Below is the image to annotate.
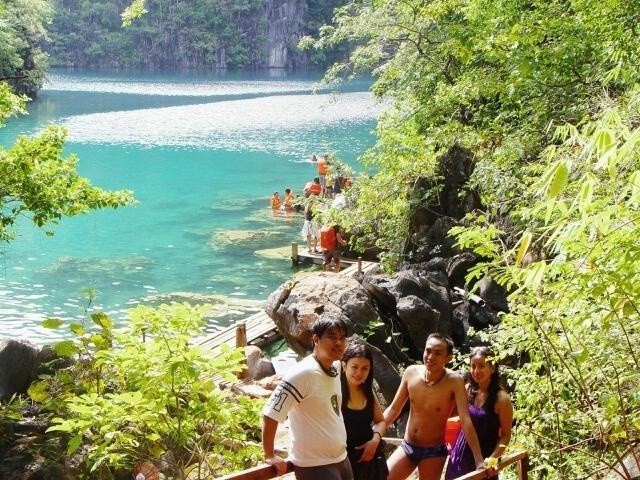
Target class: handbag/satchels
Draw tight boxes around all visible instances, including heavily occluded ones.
[372,440,386,461]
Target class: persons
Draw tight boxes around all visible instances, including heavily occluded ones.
[381,331,499,479]
[261,310,359,480]
[301,204,323,254]
[339,342,391,480]
[269,153,375,213]
[322,223,348,272]
[444,345,515,479]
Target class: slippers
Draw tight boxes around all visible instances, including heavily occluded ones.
[308,249,320,254]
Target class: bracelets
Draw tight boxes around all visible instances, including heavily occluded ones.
[373,432,383,440]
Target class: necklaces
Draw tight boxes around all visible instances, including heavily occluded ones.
[424,368,447,388]
